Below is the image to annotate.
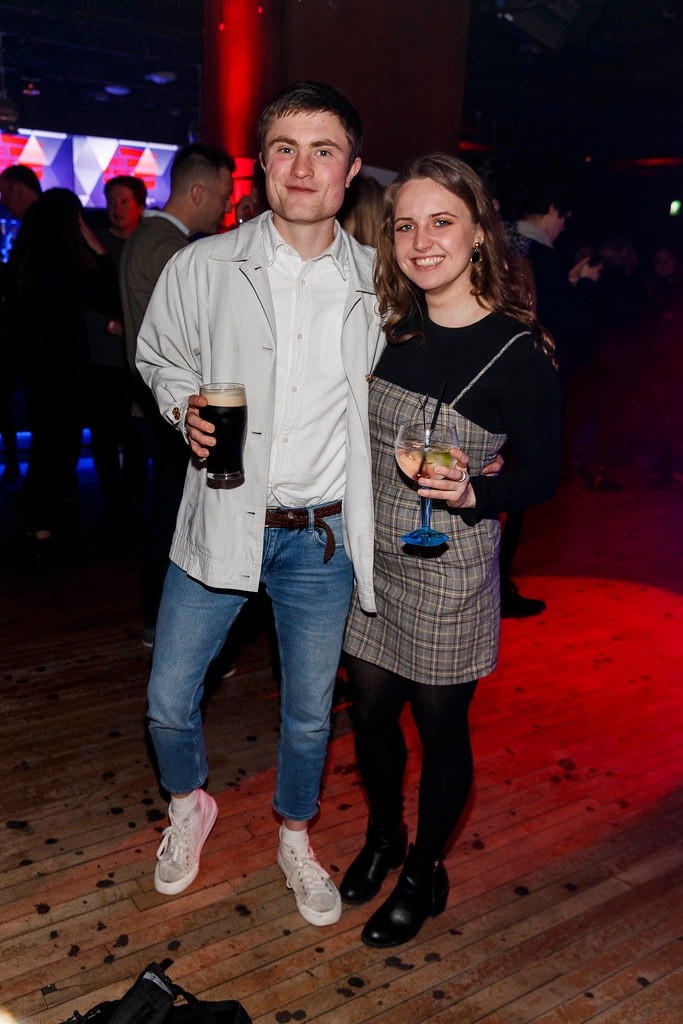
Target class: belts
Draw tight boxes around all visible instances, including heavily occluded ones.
[265,500,342,564]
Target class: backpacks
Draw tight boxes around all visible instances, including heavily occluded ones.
[60,961,253,1024]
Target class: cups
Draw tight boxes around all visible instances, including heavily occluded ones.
[200,382,247,481]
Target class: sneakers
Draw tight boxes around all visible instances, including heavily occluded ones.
[277,826,343,926]
[154,789,218,895]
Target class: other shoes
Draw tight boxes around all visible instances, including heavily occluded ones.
[499,593,545,618]
[143,626,154,647]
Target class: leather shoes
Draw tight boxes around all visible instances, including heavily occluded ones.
[361,841,450,948]
[339,824,408,907]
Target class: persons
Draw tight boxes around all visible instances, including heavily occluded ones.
[337,152,563,950]
[134,81,504,927]
[1,143,682,618]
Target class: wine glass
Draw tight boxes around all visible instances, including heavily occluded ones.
[394,419,459,545]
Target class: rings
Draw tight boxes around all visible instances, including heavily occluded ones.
[458,470,466,484]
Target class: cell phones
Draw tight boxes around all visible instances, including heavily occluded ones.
[587,255,605,266]
